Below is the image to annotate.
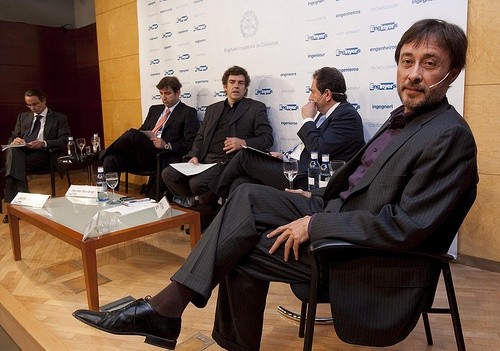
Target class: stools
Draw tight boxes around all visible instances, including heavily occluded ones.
[0,155,71,211]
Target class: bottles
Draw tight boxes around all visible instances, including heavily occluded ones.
[97,166,109,201]
[67,136,77,156]
[307,151,331,190]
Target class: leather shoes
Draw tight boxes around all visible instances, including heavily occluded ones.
[72,295,181,350]
[185,221,211,235]
[172,196,218,214]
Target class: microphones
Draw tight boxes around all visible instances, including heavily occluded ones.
[241,90,246,95]
[40,104,43,110]
[170,97,176,100]
[429,71,451,89]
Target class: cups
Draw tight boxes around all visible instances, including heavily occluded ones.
[330,161,345,176]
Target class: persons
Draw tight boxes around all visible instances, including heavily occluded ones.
[0,87,70,223]
[161,64,274,234]
[72,18,480,350]
[81,76,200,190]
[173,67,366,216]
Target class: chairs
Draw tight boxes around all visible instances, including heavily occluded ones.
[300,186,477,351]
[123,150,173,201]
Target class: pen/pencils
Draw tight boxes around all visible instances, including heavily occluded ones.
[266,149,271,155]
[120,195,151,203]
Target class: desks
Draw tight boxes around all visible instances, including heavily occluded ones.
[54,152,100,190]
[7,190,201,314]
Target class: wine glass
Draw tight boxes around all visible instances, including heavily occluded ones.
[105,172,120,204]
[283,159,298,190]
[76,137,100,157]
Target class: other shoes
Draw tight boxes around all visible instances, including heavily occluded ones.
[4,214,21,223]
[5,178,25,203]
[82,151,101,163]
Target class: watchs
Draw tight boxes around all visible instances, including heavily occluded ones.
[164,142,169,150]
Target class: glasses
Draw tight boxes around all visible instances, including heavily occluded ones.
[25,102,41,107]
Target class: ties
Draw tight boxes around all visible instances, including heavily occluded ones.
[25,115,43,143]
[152,109,170,137]
